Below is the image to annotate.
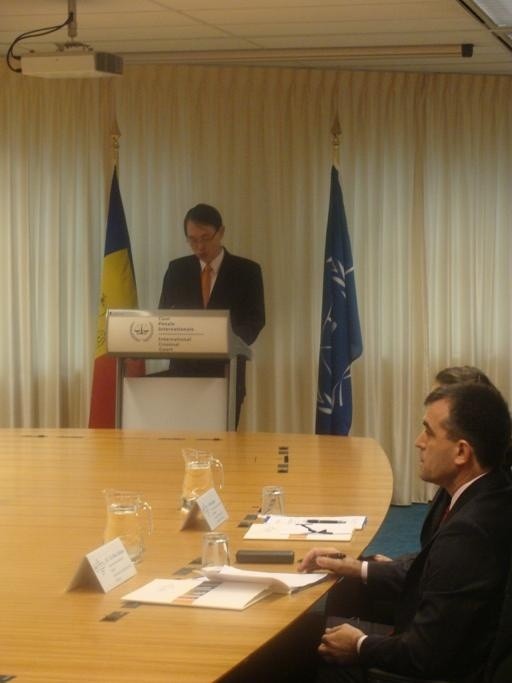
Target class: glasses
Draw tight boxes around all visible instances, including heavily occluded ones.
[183,228,218,249]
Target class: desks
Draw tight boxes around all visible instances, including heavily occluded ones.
[0,425,395,682]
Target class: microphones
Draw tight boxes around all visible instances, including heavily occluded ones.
[169,256,200,309]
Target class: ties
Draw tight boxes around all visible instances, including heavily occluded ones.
[200,264,214,307]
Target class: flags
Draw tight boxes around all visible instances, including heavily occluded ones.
[87,162,146,429]
[315,165,363,436]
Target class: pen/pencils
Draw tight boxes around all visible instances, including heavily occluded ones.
[307,519,346,523]
[297,552,347,563]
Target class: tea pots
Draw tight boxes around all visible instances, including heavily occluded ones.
[181,446,225,516]
[100,486,154,566]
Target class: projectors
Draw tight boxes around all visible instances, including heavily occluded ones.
[21,50,124,79]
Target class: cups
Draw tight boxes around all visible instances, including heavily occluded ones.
[261,484,286,519]
[203,529,230,572]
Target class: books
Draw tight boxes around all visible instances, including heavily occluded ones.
[120,512,367,612]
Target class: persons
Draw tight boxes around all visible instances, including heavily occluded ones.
[156,202,265,433]
[296,384,512,683]
[361,365,497,572]
